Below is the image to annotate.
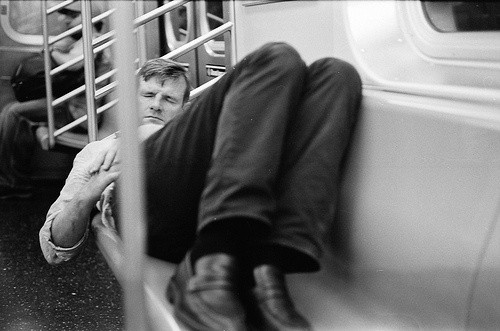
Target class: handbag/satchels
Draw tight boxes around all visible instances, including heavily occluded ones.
[10,52,72,103]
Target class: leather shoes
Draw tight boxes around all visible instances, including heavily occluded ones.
[166,242,250,331]
[252,264,315,331]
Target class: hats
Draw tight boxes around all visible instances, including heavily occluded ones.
[57,1,103,21]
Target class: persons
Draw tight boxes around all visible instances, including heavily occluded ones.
[39,41,363,331]
[0,0,108,198]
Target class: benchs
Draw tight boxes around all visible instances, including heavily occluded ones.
[35,90,117,150]
[92,82,500,330]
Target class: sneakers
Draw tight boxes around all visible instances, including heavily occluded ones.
[0,179,32,200]
